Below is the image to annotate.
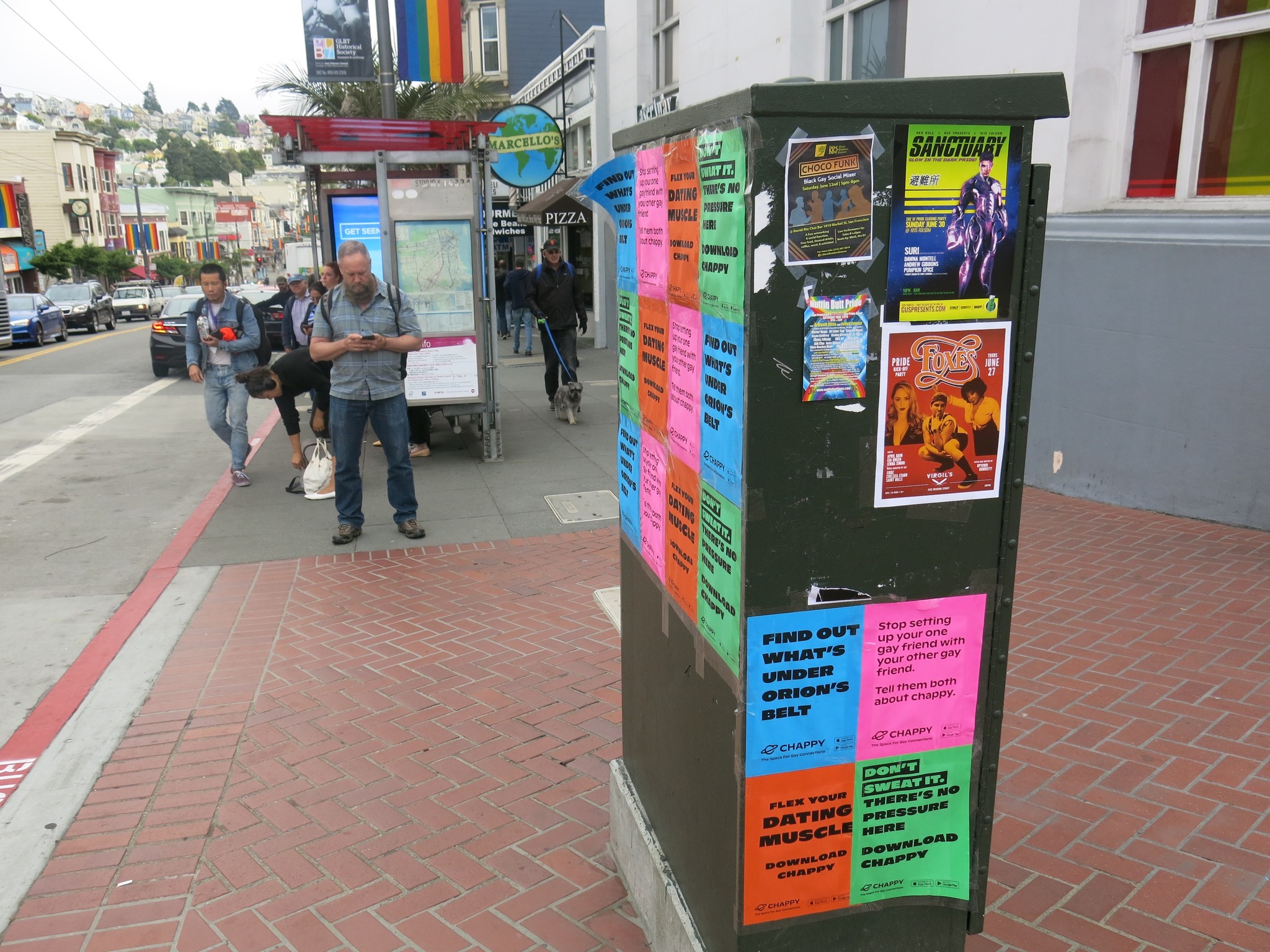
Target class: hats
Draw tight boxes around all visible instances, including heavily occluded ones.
[288,274,304,284]
[543,239,560,251]
[276,276,287,284]
[514,257,524,267]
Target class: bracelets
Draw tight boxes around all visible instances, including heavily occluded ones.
[379,336,387,350]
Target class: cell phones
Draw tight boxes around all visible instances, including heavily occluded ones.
[302,325,309,331]
[362,335,375,340]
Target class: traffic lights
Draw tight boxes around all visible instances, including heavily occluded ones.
[256,256,262,265]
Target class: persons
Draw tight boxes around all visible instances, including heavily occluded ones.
[886,379,1000,489]
[947,153,1006,296]
[309,242,424,543]
[185,239,589,486]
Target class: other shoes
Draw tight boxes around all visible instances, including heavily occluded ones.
[500,334,507,340]
[550,399,556,411]
[497,331,500,336]
[514,350,519,354]
[408,447,430,457]
[506,332,511,337]
[525,351,531,356]
[372,441,383,447]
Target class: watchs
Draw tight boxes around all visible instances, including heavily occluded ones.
[933,429,939,432]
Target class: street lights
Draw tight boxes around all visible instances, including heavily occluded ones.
[203,199,219,260]
[132,160,152,280]
[256,214,267,277]
[235,214,249,285]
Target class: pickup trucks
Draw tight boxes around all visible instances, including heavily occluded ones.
[111,279,165,323]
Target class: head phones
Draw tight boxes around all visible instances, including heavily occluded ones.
[245,379,277,391]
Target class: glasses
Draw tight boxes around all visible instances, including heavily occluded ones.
[549,250,559,254]
[310,297,320,301]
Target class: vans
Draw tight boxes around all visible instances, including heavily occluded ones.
[284,238,322,282]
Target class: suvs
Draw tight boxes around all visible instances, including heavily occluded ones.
[45,279,117,334]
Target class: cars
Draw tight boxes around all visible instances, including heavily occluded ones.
[7,292,68,348]
[149,293,272,378]
[153,284,188,306]
[186,283,287,349]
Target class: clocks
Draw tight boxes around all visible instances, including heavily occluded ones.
[72,200,87,216]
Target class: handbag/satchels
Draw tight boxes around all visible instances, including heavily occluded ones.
[303,437,332,494]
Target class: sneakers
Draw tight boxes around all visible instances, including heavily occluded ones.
[231,470,252,487]
[397,519,425,539]
[331,524,362,545]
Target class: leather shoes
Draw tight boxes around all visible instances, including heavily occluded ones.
[304,491,337,499]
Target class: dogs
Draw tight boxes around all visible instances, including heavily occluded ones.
[554,381,584,425]
